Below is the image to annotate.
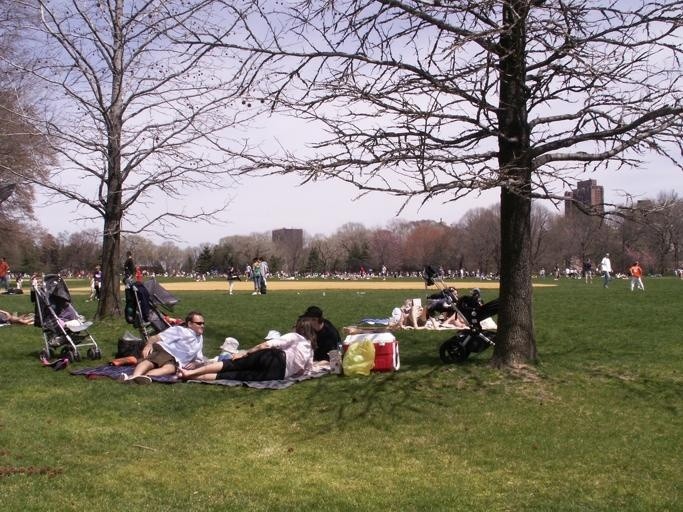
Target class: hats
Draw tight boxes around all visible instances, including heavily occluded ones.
[298,306,322,317]
[265,329,280,341]
[220,338,239,354]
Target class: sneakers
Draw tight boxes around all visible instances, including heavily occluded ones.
[119,372,151,386]
[251,292,261,295]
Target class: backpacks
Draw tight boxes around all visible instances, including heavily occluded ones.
[254,265,260,276]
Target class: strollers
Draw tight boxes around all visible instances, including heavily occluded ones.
[123,277,185,342]
[30,274,100,364]
[420,263,499,364]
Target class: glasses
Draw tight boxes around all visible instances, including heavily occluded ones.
[193,321,206,326]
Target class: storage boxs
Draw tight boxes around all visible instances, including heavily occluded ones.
[343,332,400,372]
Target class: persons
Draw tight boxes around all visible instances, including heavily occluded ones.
[539,266,545,278]
[380,264,387,281]
[176,316,319,383]
[304,305,340,366]
[388,287,484,329]
[244,257,269,295]
[89,263,102,301]
[553,256,593,285]
[628,261,644,291]
[600,252,614,289]
[115,310,206,385]
[124,250,142,289]
[0,257,39,292]
[227,267,237,295]
